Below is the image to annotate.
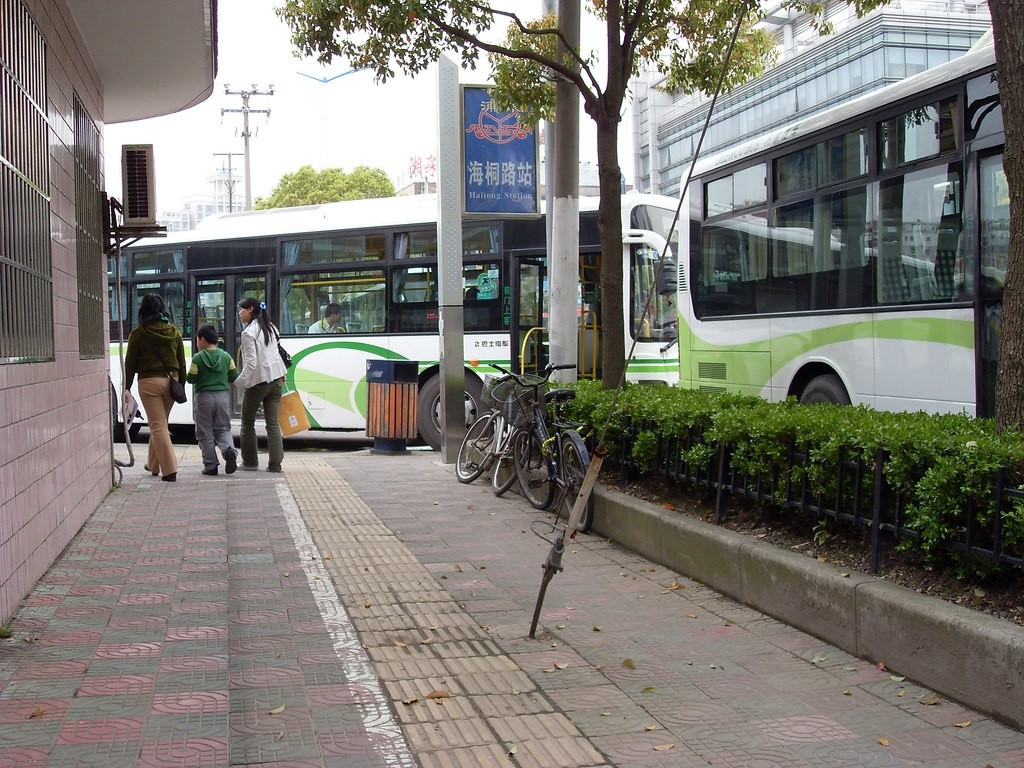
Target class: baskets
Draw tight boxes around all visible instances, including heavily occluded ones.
[480,374,514,408]
[490,377,546,428]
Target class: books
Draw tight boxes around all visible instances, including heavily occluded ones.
[119,390,138,429]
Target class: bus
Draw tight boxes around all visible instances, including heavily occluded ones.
[674,32,1015,421]
[107,188,683,448]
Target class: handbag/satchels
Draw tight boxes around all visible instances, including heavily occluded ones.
[279,383,311,438]
[270,323,292,368]
[170,375,187,404]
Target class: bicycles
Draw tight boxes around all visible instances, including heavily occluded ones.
[487,361,594,536]
[455,374,539,497]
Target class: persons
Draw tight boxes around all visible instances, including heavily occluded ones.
[466,289,477,300]
[307,304,342,333]
[126,292,186,482]
[186,325,238,475]
[231,297,288,472]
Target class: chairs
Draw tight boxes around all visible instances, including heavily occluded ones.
[293,323,310,334]
[169,304,243,334]
[860,232,868,265]
[373,325,385,332]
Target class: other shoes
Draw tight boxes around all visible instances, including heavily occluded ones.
[202,467,218,475]
[225,446,237,474]
[162,472,176,482]
[266,465,281,472]
[236,463,259,471]
[144,463,158,476]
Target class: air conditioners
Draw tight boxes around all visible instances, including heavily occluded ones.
[120,143,158,225]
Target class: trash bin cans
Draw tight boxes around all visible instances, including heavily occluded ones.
[364,358,421,455]
